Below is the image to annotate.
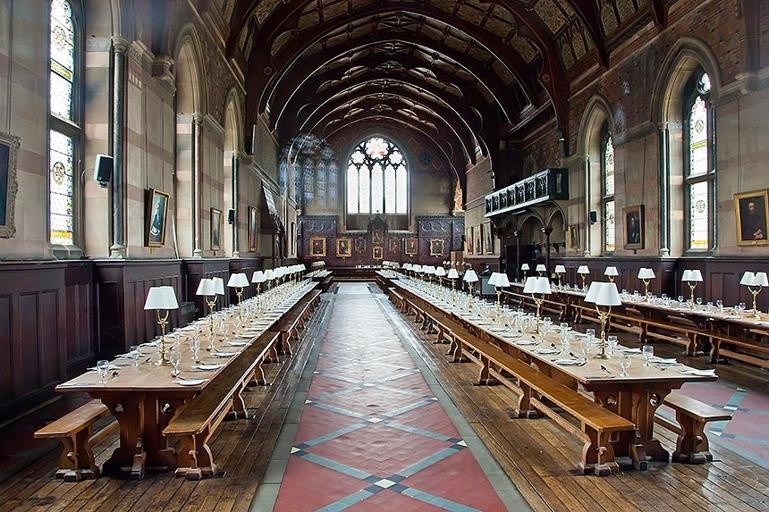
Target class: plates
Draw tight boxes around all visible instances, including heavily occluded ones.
[508,280,769,328]
[394,280,676,367]
[88,281,316,385]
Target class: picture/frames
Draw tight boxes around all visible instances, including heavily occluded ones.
[733,188,769,247]
[404,237,418,255]
[484,220,494,254]
[0,131,23,239]
[465,226,473,255]
[309,237,326,257]
[568,223,580,249]
[145,187,170,247]
[429,239,445,257]
[335,238,352,258]
[248,206,260,252]
[622,204,645,250]
[474,223,482,255]
[211,207,222,251]
[372,246,383,259]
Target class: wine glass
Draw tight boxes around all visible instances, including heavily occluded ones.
[513,278,761,322]
[97,277,312,387]
[398,275,654,376]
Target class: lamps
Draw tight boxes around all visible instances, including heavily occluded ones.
[143,259,327,366]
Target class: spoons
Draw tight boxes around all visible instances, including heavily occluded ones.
[103,281,311,384]
[402,282,669,373]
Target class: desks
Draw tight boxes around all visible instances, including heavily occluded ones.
[325,266,381,278]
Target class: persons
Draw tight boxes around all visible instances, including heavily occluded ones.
[372,229,384,243]
[740,199,768,240]
[406,239,416,254]
[388,238,400,254]
[432,241,443,254]
[626,216,640,244]
[355,239,364,251]
[339,241,348,254]
[151,203,164,235]
[312,241,323,254]
[374,249,381,257]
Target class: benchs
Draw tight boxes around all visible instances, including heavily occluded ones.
[322,276,334,292]
[162,329,281,480]
[34,397,120,482]
[375,275,385,289]
[452,328,636,477]
[653,389,732,464]
